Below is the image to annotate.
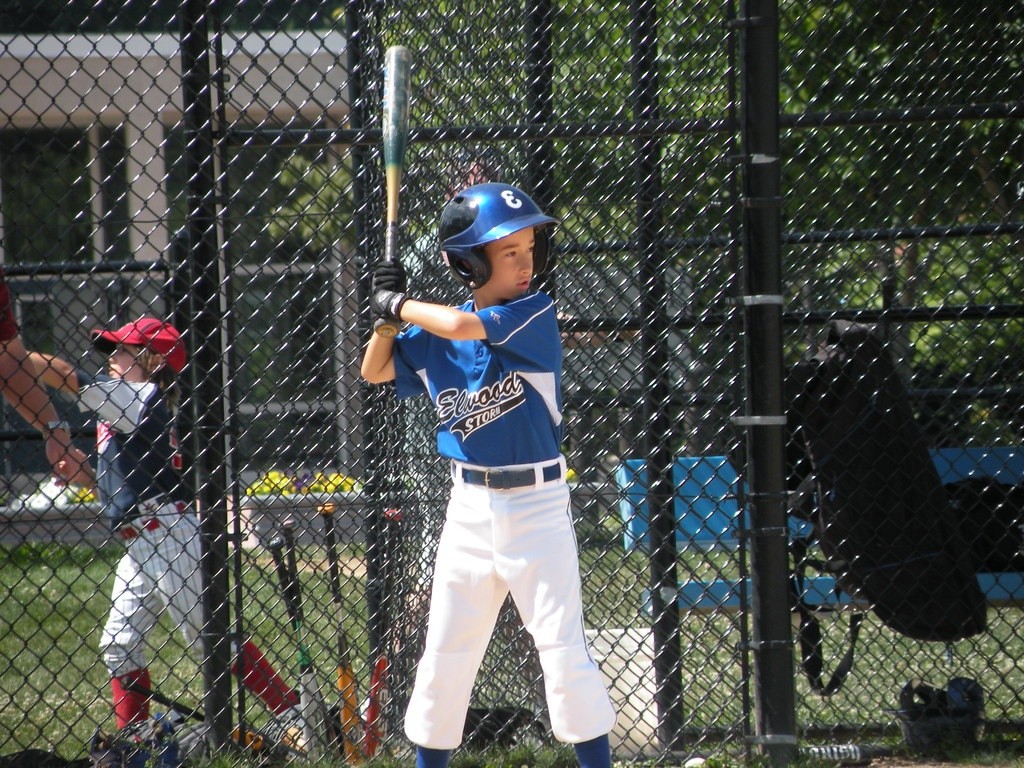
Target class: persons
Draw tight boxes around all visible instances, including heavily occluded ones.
[362,183,618,768]
[27,318,306,750]
[0,278,94,488]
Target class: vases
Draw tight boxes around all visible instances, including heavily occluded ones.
[0,502,113,546]
[236,496,424,546]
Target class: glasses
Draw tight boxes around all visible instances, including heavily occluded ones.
[116,345,136,359]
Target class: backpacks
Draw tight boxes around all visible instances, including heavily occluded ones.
[778,326,989,695]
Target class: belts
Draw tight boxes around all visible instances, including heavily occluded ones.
[120,503,187,540]
[453,463,561,490]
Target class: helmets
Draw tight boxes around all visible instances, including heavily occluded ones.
[891,677,987,760]
[438,182,560,289]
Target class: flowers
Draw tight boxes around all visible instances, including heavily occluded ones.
[12,478,95,510]
[246,470,414,496]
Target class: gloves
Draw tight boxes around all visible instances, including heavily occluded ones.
[371,259,407,322]
[1,265,16,342]
[370,289,413,322]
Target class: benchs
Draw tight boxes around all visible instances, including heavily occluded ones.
[616,446,1024,616]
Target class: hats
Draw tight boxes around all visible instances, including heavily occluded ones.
[91,318,186,375]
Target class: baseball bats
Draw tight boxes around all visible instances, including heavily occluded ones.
[116,675,291,763]
[798,743,876,767]
[266,515,343,767]
[315,501,367,767]
[362,508,407,760]
[372,43,415,340]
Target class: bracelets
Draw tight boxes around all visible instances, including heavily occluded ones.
[44,421,71,437]
